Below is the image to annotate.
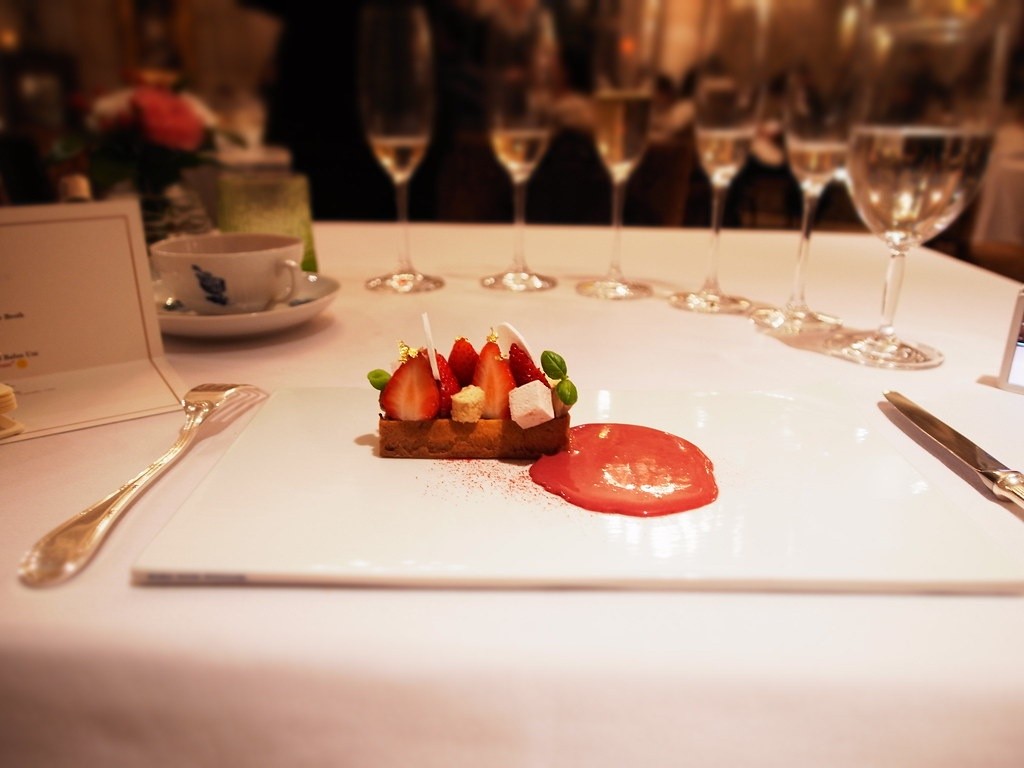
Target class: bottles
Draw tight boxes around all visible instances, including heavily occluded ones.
[214,147,318,273]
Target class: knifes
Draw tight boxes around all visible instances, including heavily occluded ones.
[882,389,1024,510]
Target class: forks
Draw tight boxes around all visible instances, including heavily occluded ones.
[17,382,271,591]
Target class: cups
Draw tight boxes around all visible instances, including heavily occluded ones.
[149,233,304,314]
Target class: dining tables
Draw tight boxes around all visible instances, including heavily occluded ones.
[0,220,1024,768]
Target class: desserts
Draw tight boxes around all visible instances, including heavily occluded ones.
[367,312,578,459]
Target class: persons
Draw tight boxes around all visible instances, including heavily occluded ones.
[0,0,992,262]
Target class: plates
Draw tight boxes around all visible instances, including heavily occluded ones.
[132,365,1022,600]
[151,271,341,337]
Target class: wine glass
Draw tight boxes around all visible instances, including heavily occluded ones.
[575,0,664,302]
[481,2,557,290]
[355,3,444,294]
[664,0,1011,369]
